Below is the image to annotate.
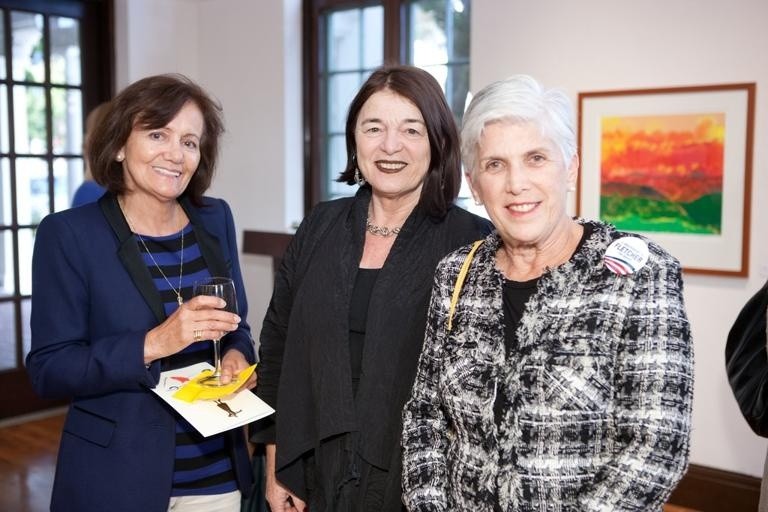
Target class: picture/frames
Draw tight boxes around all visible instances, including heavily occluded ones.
[575,82,754,278]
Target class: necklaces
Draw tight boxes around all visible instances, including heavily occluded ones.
[366,217,402,237]
[118,195,186,308]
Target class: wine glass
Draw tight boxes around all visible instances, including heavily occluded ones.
[192,277,239,388]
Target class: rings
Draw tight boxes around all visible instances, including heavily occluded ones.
[193,329,202,340]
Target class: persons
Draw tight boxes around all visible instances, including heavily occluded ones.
[399,74,697,512]
[250,64,496,511]
[25,73,261,510]
[70,104,117,207]
